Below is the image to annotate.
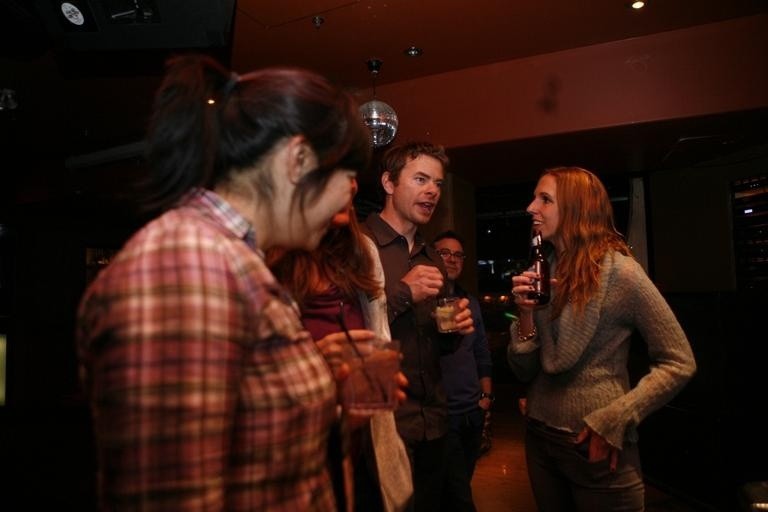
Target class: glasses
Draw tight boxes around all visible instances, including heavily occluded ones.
[437,248,464,260]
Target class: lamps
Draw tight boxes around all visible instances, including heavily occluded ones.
[63,3,149,27]
[352,59,398,147]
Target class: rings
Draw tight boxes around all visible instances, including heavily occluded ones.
[509,289,518,297]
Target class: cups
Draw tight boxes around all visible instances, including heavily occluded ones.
[339,338,402,411]
[433,298,459,335]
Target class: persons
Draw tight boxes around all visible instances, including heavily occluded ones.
[505,166,698,512]
[72,54,410,510]
[353,139,474,511]
[254,138,408,512]
[426,230,495,510]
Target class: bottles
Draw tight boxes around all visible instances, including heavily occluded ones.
[526,230,552,305]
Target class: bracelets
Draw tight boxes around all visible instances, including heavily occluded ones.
[479,392,495,401]
[512,319,537,341]
